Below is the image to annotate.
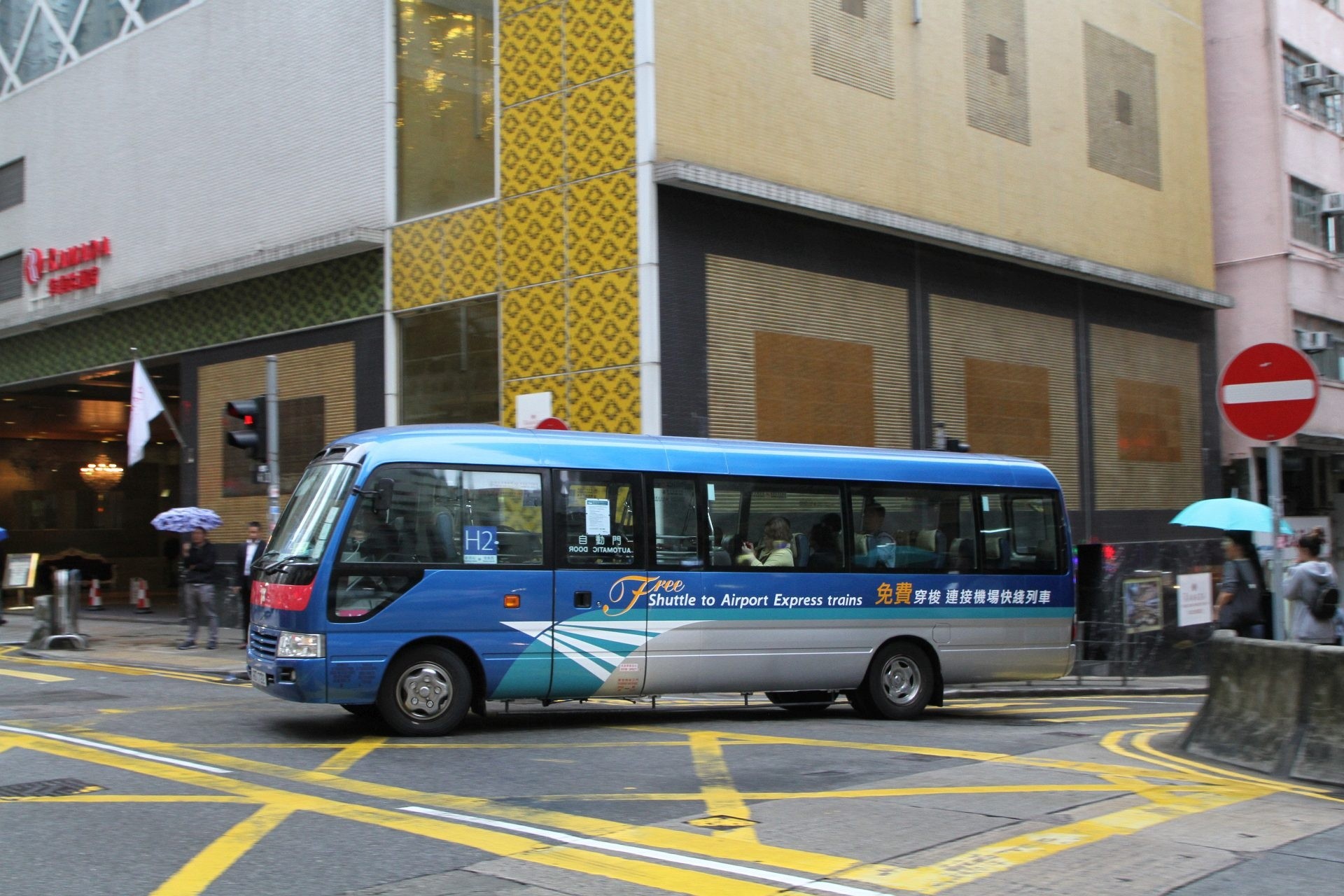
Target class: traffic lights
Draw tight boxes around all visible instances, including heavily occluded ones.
[222,396,264,460]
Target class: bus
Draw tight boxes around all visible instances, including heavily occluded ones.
[239,423,1078,732]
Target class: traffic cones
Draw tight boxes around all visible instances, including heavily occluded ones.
[132,577,153,616]
[87,573,103,610]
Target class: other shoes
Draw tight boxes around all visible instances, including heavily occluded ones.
[239,643,247,650]
[179,639,197,650]
[205,641,218,650]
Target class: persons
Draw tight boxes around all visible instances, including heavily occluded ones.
[345,505,409,592]
[233,521,266,650]
[1212,532,1264,639]
[735,502,896,572]
[177,526,218,650]
[1281,526,1341,644]
[656,527,732,566]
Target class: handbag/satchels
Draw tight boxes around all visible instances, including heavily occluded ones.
[1218,560,1265,630]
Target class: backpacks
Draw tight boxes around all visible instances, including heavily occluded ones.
[1298,583,1340,621]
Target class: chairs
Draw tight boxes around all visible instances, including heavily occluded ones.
[711,519,1051,566]
[427,506,459,561]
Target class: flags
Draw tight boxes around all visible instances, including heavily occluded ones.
[127,359,165,466]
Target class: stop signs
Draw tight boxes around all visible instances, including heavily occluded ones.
[1213,338,1317,447]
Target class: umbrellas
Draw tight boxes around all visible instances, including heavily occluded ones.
[1168,496,1293,535]
[150,506,224,553]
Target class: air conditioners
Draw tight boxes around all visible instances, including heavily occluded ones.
[1318,72,1344,96]
[1299,331,1330,351]
[1323,193,1344,214]
[1299,62,1327,86]
